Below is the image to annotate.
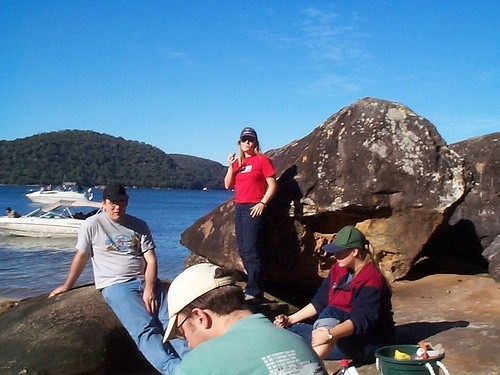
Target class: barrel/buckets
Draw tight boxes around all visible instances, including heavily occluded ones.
[374,345,445,375]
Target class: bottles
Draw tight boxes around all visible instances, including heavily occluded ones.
[336,359,350,375]
[411,348,427,360]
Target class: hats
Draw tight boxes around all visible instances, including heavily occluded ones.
[162,263,235,344]
[322,225,365,252]
[240,127,257,139]
[103,181,129,202]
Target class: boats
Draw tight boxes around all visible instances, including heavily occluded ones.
[0,200,105,237]
[25,182,103,208]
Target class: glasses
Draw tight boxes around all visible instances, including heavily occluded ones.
[105,199,128,207]
[174,310,195,340]
[240,138,255,142]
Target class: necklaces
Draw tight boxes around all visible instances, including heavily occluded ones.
[259,202,266,205]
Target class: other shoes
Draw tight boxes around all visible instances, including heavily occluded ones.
[242,289,258,301]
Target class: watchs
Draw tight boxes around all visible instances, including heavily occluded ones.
[327,330,333,341]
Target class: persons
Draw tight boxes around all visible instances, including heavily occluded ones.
[273,225,395,363]
[162,262,331,375]
[5,207,21,218]
[48,182,192,375]
[224,127,278,299]
